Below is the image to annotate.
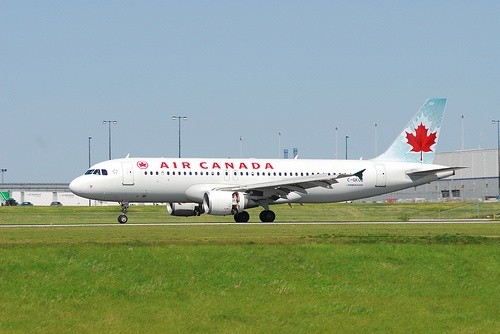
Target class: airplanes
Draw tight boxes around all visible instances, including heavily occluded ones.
[67,98,471,225]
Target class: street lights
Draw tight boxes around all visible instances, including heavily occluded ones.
[1,168,7,184]
[239,137,243,159]
[374,122,378,156]
[335,127,338,158]
[172,116,188,158]
[492,119,500,199]
[344,135,349,160]
[102,121,117,160]
[335,127,339,158]
[88,136,92,168]
[279,132,281,158]
[460,113,464,151]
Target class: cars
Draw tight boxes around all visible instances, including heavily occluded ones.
[50,201,62,206]
[21,201,33,206]
